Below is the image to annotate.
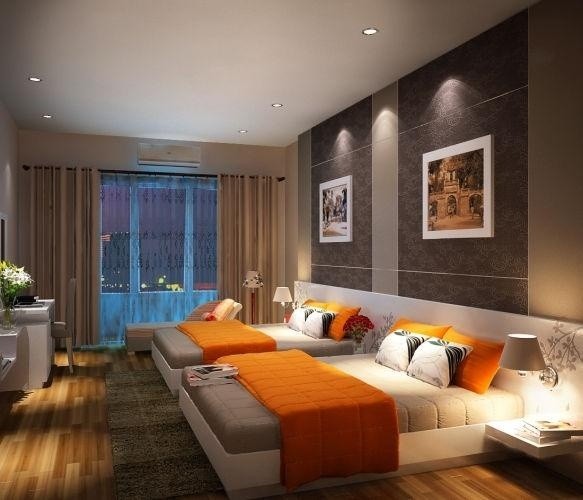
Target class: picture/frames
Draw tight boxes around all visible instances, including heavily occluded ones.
[317,175,354,245]
[420,133,493,241]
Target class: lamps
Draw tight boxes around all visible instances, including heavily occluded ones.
[272,286,299,310]
[240,270,263,326]
[497,332,556,391]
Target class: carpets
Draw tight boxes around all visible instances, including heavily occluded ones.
[101,367,228,500]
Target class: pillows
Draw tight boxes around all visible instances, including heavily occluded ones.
[286,305,315,332]
[373,327,429,372]
[186,298,235,323]
[389,317,451,339]
[302,298,328,309]
[325,301,360,342]
[442,325,505,395]
[302,310,337,338]
[408,334,474,389]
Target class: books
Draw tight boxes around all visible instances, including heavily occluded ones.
[516,415,582,445]
[188,381,233,386]
[187,362,238,379]
[186,371,236,383]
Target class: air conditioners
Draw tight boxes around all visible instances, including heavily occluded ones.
[136,142,202,169]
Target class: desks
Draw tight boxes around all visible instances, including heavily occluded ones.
[0,297,55,390]
[0,327,23,383]
[486,418,582,460]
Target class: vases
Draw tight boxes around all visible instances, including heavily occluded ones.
[352,335,365,354]
[1,305,18,330]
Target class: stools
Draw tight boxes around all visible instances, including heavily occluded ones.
[50,322,76,375]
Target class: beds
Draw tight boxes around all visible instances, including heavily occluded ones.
[175,296,582,500]
[124,294,241,354]
[150,281,378,397]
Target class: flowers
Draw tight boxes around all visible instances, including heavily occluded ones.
[340,312,373,342]
[0,262,34,329]
